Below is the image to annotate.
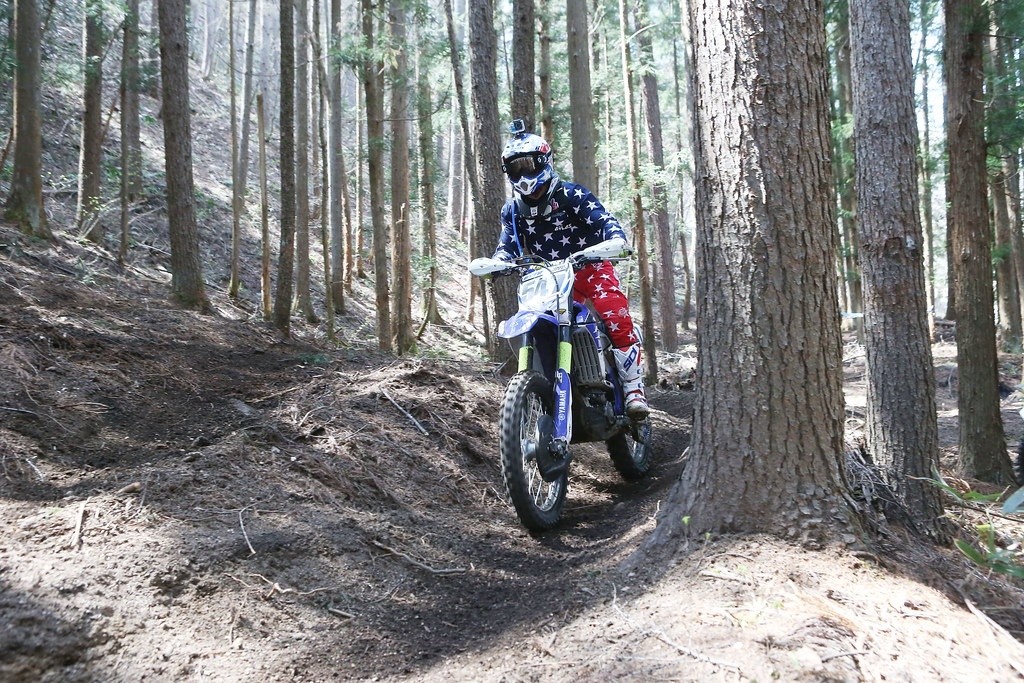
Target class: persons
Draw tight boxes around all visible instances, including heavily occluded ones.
[478,133,651,421]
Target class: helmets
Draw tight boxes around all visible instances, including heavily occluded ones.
[501,132,555,196]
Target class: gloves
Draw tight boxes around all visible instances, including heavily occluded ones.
[609,249,628,267]
[480,273,495,279]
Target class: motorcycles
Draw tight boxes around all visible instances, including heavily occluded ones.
[467,239,654,533]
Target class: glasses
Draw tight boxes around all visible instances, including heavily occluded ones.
[500,147,553,182]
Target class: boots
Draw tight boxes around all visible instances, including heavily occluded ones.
[612,342,650,421]
